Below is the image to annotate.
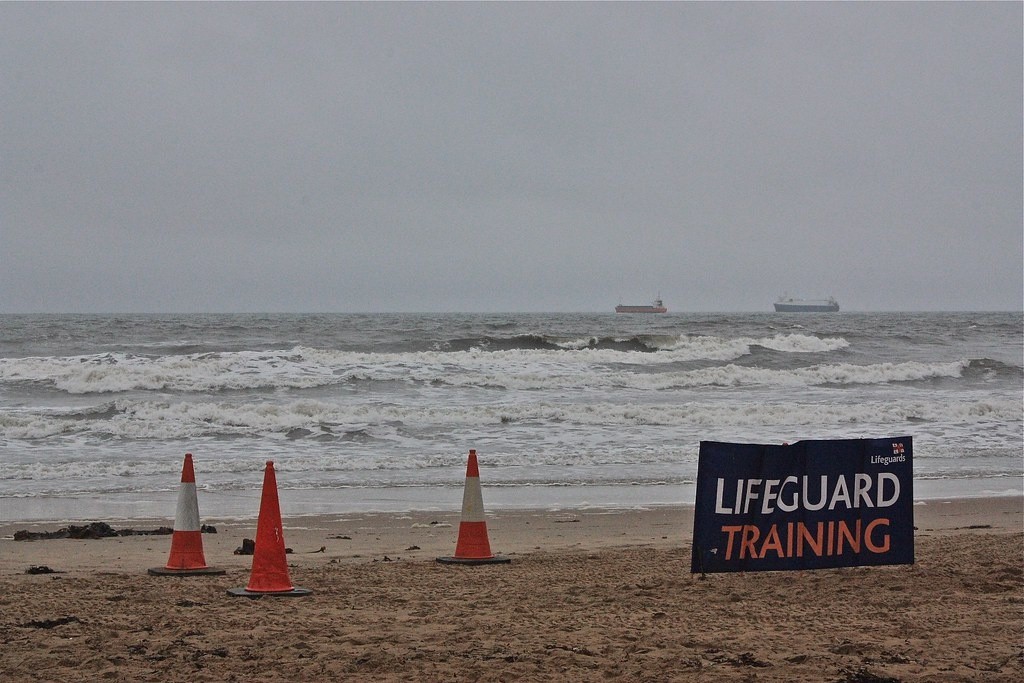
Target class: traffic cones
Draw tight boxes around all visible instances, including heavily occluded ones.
[434,449,512,566]
[227,461,306,598]
[146,453,226,576]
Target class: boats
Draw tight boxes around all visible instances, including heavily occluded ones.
[615,300,669,317]
[773,292,839,313]
